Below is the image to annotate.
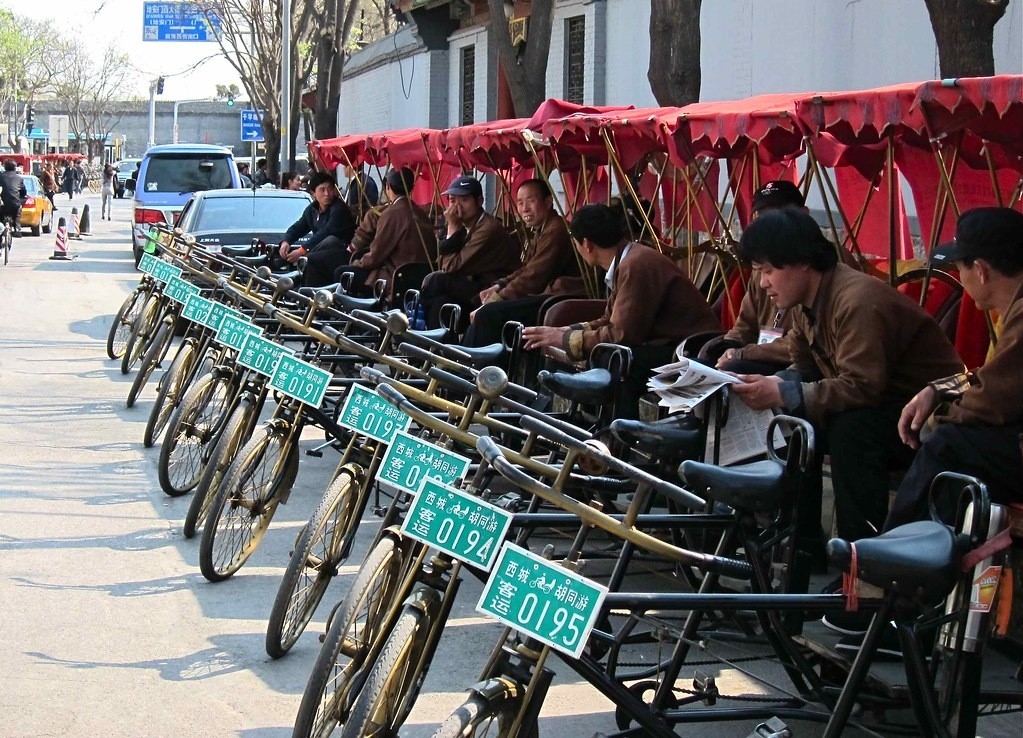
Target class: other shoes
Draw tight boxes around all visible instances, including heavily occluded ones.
[52,207,58,211]
[800,534,827,563]
[13,232,22,237]
[822,611,875,633]
[836,633,904,659]
[108,217,111,221]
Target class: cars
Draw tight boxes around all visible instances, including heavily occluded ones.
[0,173,54,237]
[165,188,320,334]
[113,158,145,198]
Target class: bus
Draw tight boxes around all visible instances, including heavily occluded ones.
[0,127,122,186]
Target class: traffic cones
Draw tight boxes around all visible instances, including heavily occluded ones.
[50,217,78,261]
[66,207,83,240]
[79,204,94,236]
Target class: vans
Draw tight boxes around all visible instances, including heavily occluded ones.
[131,140,242,260]
[234,155,316,194]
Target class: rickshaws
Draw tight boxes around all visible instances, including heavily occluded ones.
[103,100,1019,738]
[0,154,39,177]
[41,153,88,192]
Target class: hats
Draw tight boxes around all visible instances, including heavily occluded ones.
[929,207,1023,266]
[751,179,804,215]
[441,176,481,197]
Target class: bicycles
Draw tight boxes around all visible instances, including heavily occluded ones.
[2,214,11,265]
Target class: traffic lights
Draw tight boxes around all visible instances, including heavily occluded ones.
[226,99,234,106]
[156,77,164,95]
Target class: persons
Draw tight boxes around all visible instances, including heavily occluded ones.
[278,172,356,288]
[822,206,1023,655]
[761,339,768,345]
[101,158,124,221]
[344,160,378,219]
[0,159,27,256]
[237,158,316,192]
[132,161,142,180]
[15,160,86,211]
[338,167,971,598]
